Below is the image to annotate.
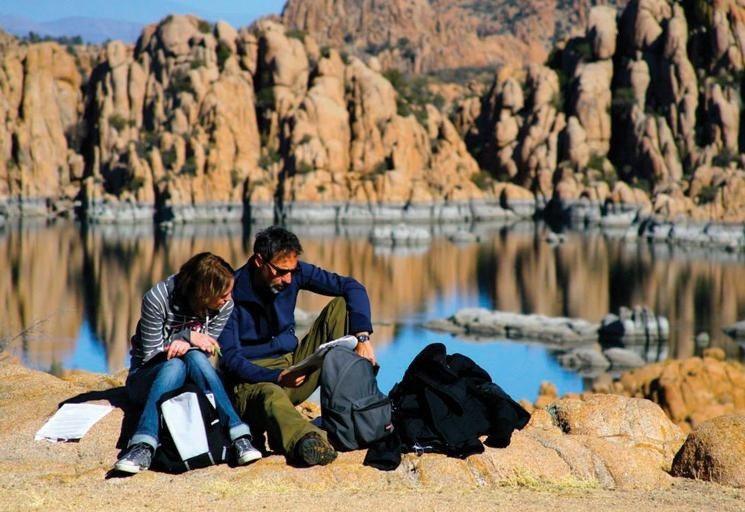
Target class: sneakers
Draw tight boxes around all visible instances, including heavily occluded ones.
[231,438,262,465]
[115,443,152,473]
[298,432,337,465]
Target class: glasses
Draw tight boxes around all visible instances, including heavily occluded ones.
[268,261,296,275]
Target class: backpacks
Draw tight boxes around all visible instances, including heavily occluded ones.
[319,345,395,451]
[156,383,230,474]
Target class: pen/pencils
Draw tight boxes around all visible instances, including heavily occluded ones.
[214,345,223,357]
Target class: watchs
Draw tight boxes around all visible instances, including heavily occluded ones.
[355,334,370,342]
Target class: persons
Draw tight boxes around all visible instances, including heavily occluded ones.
[214,225,374,466]
[113,253,263,473]
